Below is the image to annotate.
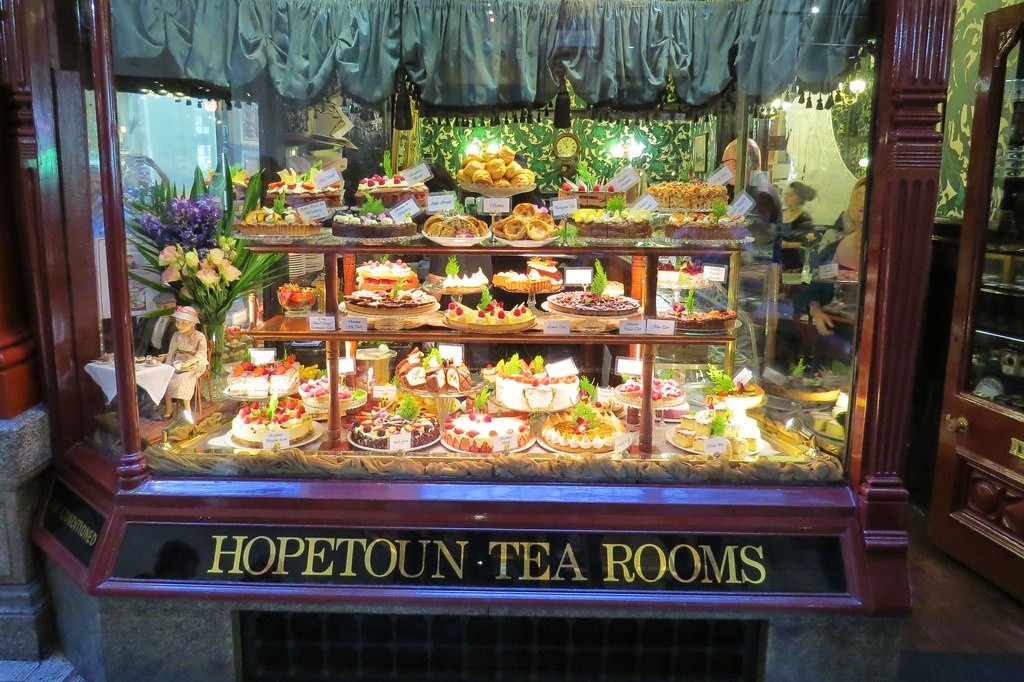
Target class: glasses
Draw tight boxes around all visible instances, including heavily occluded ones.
[720,158,736,165]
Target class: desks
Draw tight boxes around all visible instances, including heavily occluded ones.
[84,359,175,421]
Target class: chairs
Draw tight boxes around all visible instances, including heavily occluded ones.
[195,339,213,412]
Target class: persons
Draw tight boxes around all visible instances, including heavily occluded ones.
[424,164,494,310]
[782,181,816,273]
[720,138,782,358]
[163,306,207,418]
[800,172,867,380]
[462,155,558,358]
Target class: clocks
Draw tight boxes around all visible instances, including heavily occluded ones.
[553,132,580,160]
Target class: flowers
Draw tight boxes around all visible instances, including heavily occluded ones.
[120,165,295,362]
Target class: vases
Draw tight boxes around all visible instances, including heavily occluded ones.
[201,323,230,403]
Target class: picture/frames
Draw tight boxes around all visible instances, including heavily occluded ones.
[693,132,709,173]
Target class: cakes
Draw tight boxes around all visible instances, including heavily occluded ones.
[226,151,839,455]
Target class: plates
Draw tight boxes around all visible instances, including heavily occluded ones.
[492,234,560,247]
[421,229,492,246]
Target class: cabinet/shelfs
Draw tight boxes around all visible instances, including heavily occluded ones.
[242,240,742,453]
[927,3,1024,606]
